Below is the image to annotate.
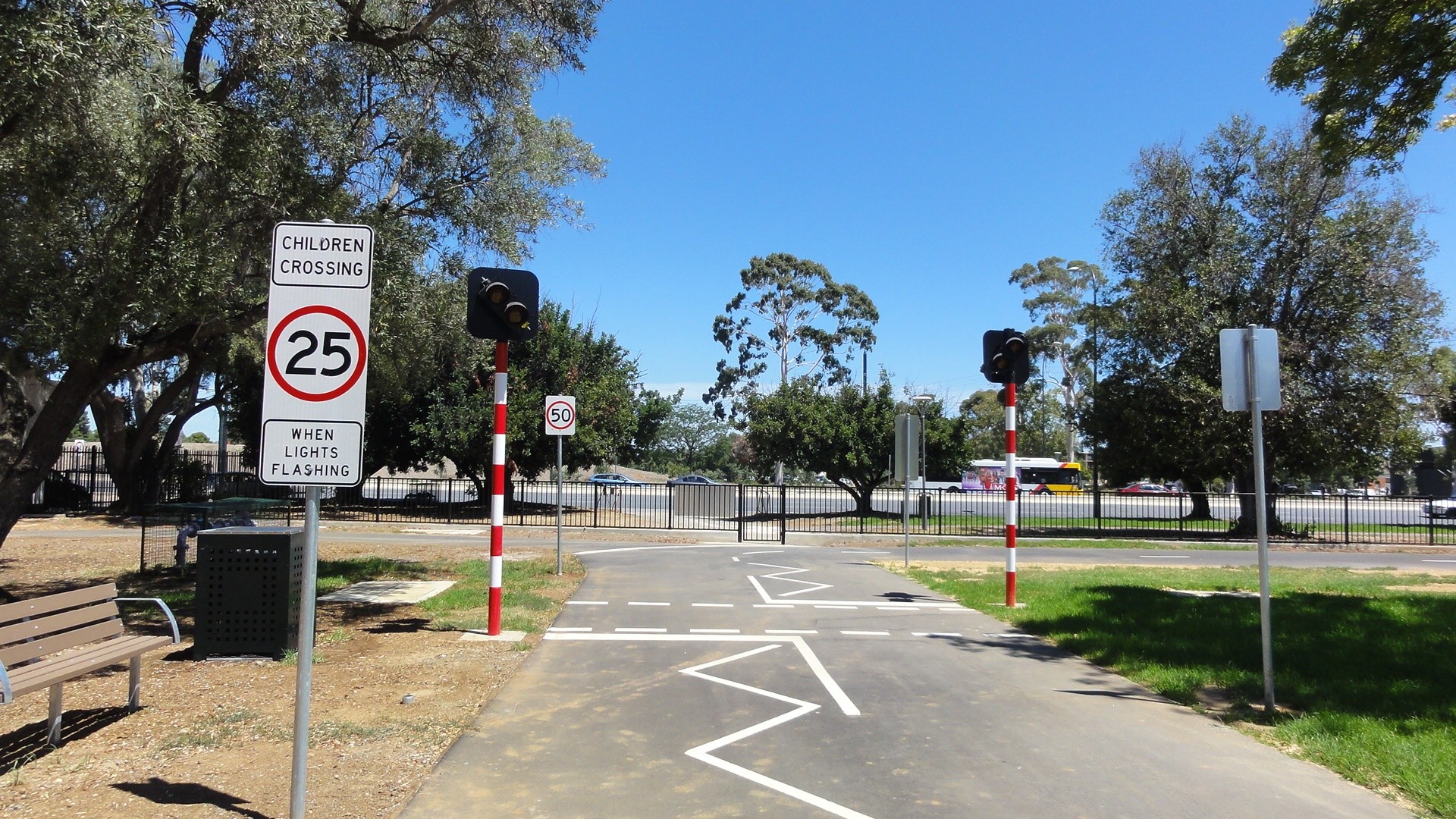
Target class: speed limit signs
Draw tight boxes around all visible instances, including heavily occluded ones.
[267,305,366,402]
[546,395,576,436]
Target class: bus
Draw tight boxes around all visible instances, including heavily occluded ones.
[961,458,1084,496]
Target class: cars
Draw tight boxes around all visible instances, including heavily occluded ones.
[1421,495,1456,519]
[667,475,720,485]
[1117,482,1187,498]
[189,472,293,500]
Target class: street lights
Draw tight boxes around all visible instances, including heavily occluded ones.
[912,397,932,530]
[1067,265,1100,518]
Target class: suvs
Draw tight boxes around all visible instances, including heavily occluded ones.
[586,473,651,487]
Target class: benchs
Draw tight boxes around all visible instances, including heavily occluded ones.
[0,582,180,746]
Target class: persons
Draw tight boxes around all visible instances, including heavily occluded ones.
[1320,483,1325,500]
[985,470,991,489]
[991,470,997,489]
[997,470,1005,484]
[1386,487,1388,496]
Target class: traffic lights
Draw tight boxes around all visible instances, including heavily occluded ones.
[466,267,540,341]
[982,329,1030,385]
